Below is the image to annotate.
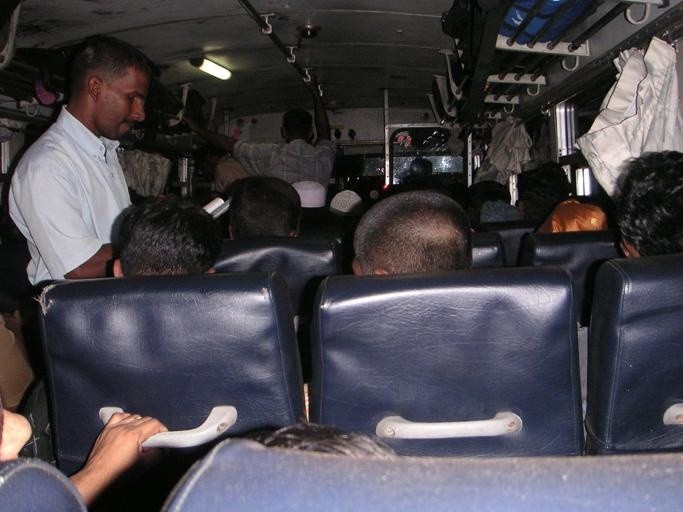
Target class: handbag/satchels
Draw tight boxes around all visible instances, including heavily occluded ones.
[0,45,183,141]
[440,0,580,77]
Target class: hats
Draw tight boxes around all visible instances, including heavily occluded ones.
[331,190,362,216]
[292,181,325,208]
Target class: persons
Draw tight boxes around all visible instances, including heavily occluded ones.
[115,149,683,438]
[181,74,336,193]
[1,304,168,512]
[263,419,399,456]
[9,35,151,286]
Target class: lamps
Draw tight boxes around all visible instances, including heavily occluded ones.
[190,56,232,85]
